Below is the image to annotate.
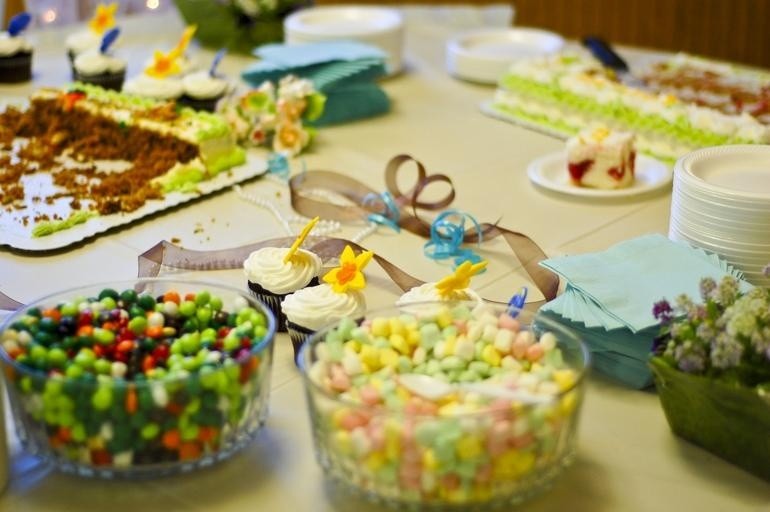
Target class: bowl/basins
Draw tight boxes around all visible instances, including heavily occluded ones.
[1,278,277,481]
[296,301,592,510]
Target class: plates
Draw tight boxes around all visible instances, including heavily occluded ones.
[284,1,407,79]
[670,147,770,289]
[446,28,566,88]
[526,149,672,200]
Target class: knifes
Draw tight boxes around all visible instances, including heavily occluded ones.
[583,34,648,91]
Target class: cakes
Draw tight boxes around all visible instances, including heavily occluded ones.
[280,244,373,369]
[0,82,246,235]
[243,217,321,332]
[183,70,231,109]
[67,34,125,93]
[0,37,36,82]
[496,34,769,161]
[560,124,642,187]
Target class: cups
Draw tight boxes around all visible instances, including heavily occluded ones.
[1,48,32,84]
[72,67,126,94]
[283,316,370,366]
[181,91,227,116]
[248,278,321,334]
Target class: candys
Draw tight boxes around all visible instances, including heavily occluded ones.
[311,302,575,506]
[0,288,267,467]
